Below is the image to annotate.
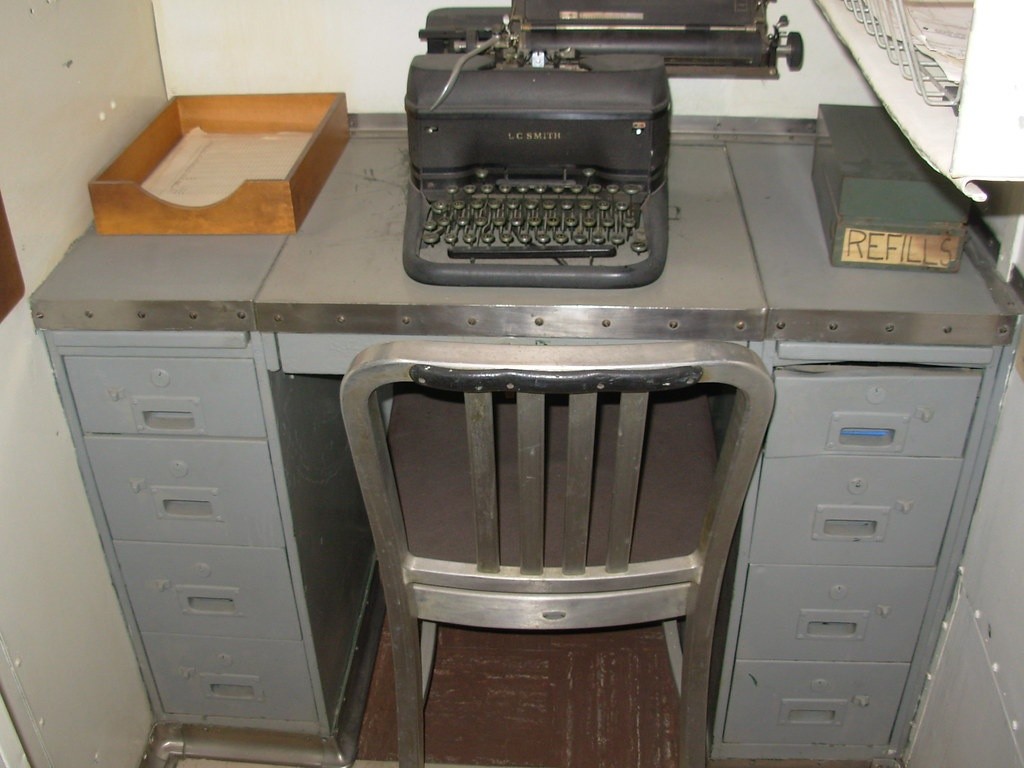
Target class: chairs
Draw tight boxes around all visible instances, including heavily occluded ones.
[338,341,775,768]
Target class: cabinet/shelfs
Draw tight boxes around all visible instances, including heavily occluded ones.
[29,127,1024,768]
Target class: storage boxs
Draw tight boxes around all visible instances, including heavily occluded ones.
[89,94,347,237]
[815,104,969,274]
